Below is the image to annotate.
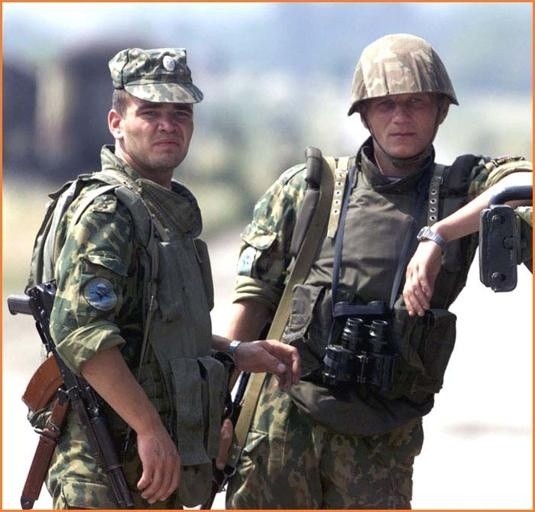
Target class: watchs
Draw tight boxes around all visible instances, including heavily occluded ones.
[414,225,447,254]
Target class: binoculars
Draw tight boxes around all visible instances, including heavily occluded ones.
[323,318,392,401]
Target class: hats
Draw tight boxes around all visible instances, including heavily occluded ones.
[109,48,203,104]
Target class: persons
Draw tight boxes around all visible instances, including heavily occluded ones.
[223,31,532,510]
[21,44,306,509]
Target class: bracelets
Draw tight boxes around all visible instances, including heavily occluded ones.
[226,339,242,356]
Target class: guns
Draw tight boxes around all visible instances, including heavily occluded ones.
[6,281,133,506]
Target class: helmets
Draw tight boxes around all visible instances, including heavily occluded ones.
[348,34,459,116]
